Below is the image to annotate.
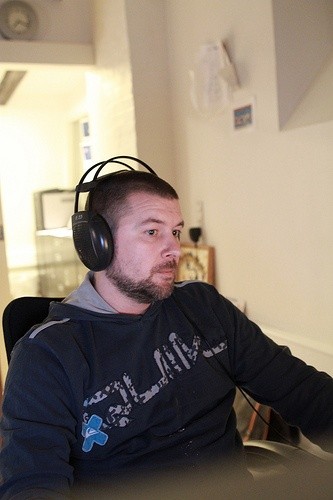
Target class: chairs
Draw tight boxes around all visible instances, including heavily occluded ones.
[3,298,331,500]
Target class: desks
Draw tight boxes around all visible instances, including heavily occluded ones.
[173,240,216,288]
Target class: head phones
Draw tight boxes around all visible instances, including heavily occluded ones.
[71,156,157,271]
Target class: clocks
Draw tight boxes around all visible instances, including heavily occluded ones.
[0,0,40,41]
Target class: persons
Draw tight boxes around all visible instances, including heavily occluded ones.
[1,171,333,500]
